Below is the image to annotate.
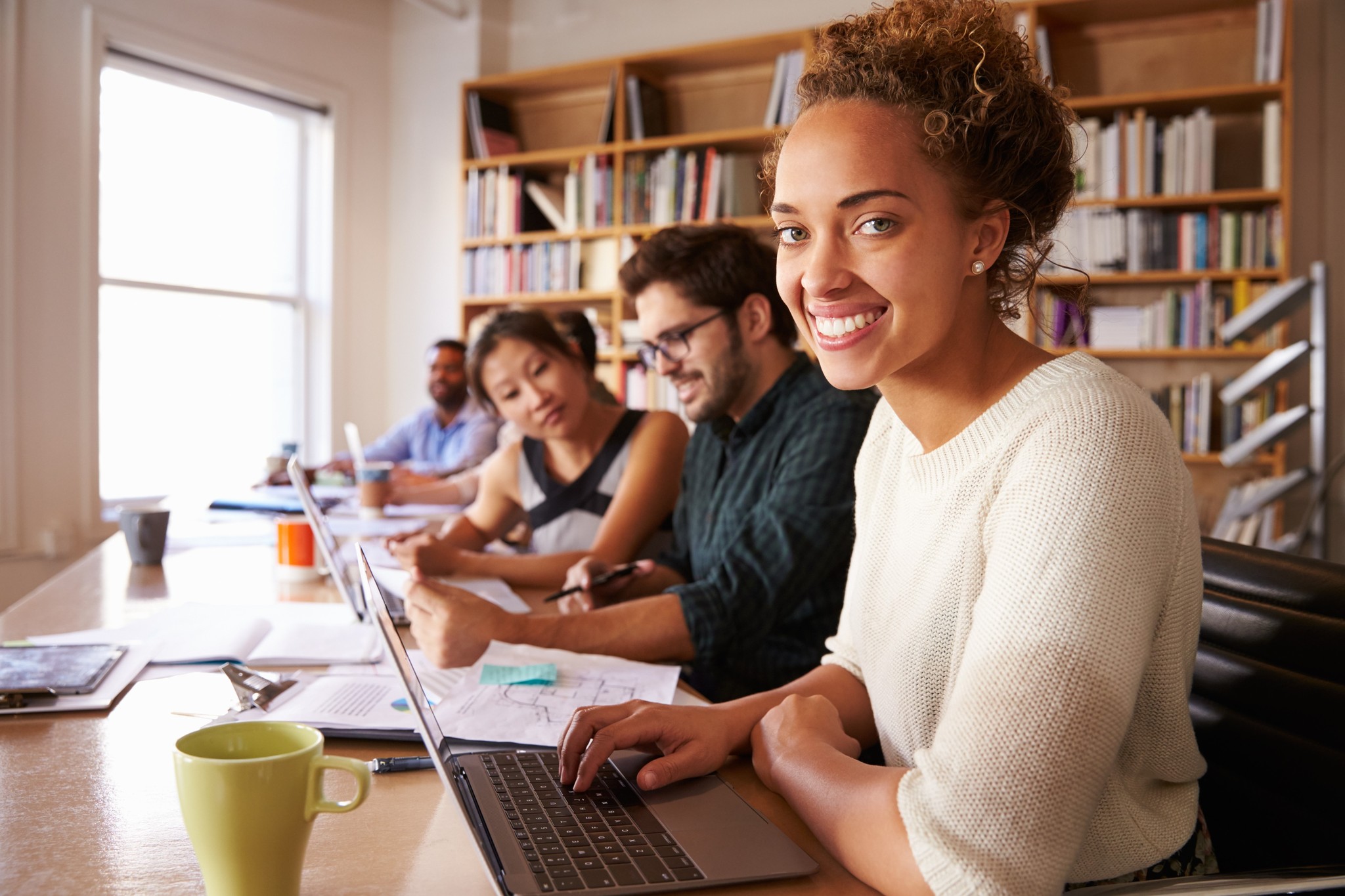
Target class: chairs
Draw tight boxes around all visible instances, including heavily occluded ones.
[1074,531,1344,896]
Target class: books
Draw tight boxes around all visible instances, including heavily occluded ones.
[465,50,815,411]
[1004,0,1286,551]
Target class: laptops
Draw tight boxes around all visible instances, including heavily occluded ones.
[286,455,816,896]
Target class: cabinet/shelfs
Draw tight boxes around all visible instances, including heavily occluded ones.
[458,2,1335,555]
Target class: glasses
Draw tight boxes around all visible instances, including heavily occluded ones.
[637,310,736,369]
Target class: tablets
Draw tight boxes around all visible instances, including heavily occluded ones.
[0,644,129,693]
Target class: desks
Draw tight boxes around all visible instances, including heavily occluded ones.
[0,487,870,896]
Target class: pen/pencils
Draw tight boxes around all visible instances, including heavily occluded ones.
[363,757,435,772]
[543,564,638,601]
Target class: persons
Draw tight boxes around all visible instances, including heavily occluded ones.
[322,309,694,584]
[404,221,879,702]
[556,0,1220,896]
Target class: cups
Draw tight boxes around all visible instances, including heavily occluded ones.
[120,510,170,564]
[274,518,333,584]
[354,461,393,518]
[174,721,370,896]
